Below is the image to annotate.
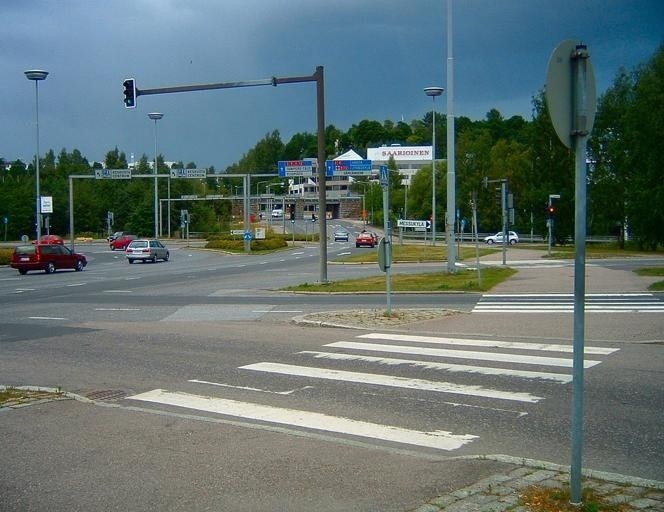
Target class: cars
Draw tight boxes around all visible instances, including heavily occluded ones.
[356,230,379,248]
[32,234,64,245]
[125,239,169,263]
[484,230,519,245]
[334,229,348,241]
[109,236,134,251]
[9,244,87,274]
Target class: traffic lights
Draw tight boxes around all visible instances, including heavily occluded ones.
[122,78,137,110]
[545,206,555,220]
[184,213,190,223]
[290,203,296,223]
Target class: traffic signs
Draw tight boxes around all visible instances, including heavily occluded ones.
[277,159,312,179]
[170,168,207,179]
[95,168,131,179]
[326,159,371,177]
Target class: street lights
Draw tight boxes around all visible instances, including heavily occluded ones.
[23,70,49,241]
[148,111,165,237]
[424,87,444,247]
